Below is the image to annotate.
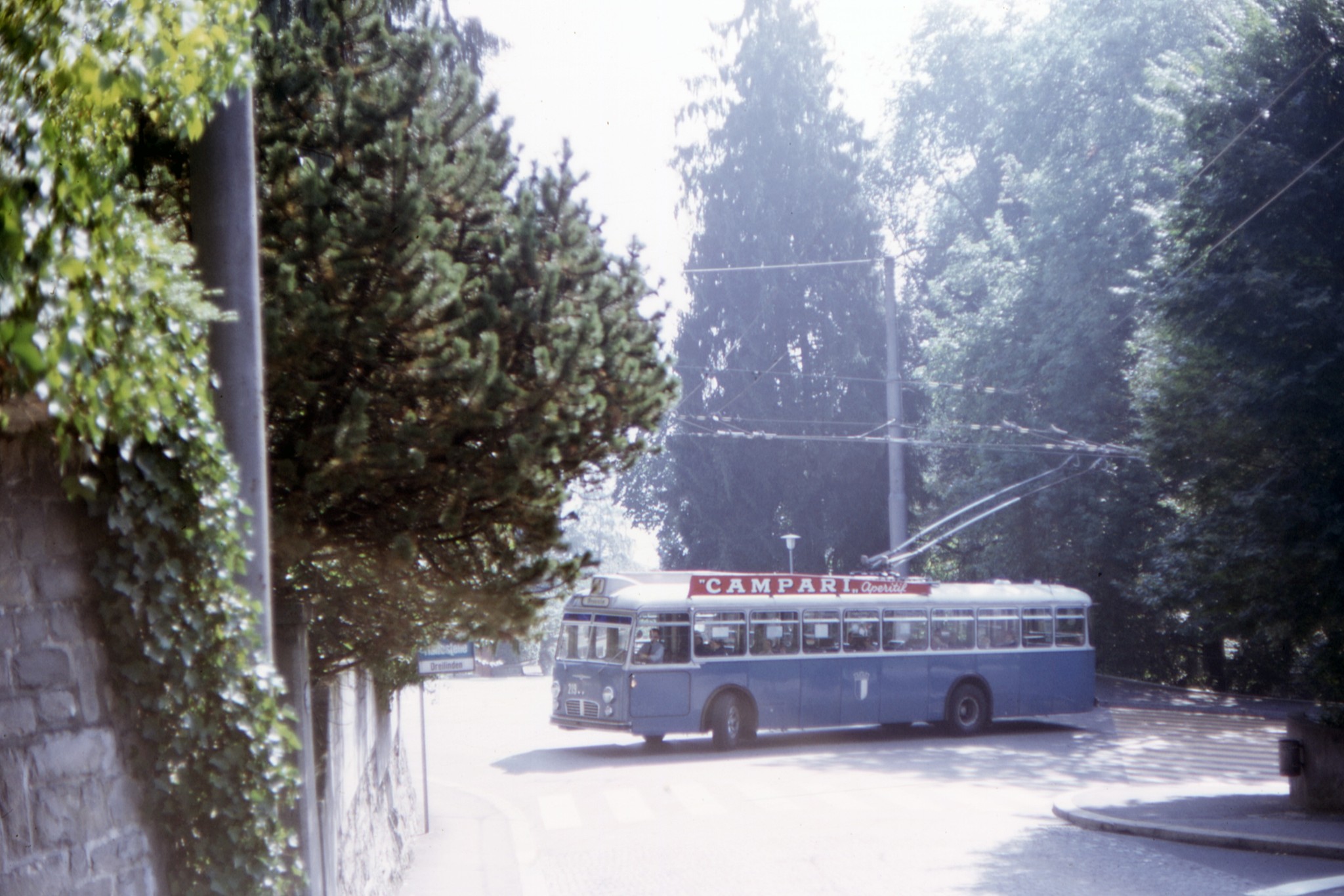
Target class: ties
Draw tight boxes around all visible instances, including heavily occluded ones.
[647,646,652,655]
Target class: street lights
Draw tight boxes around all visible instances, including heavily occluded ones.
[780,532,802,632]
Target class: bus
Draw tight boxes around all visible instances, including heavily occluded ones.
[549,572,1095,749]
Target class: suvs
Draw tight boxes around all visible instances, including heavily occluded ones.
[538,629,604,676]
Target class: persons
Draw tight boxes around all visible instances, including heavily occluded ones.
[635,629,665,665]
[750,629,772,655]
[695,632,727,655]
[803,634,823,654]
